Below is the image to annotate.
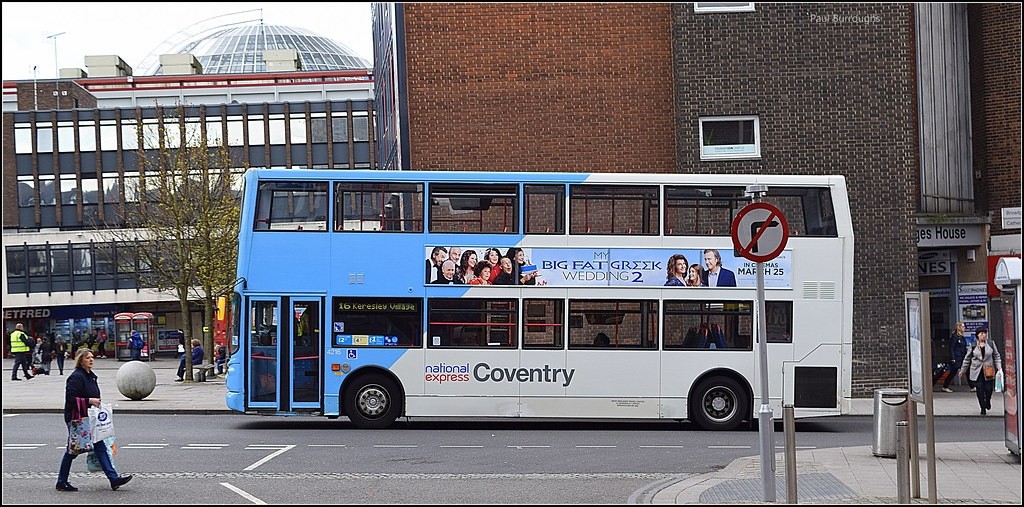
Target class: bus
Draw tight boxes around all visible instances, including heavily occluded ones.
[210,166,854,432]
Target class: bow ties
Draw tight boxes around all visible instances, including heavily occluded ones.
[448,280,454,282]
[433,264,440,269]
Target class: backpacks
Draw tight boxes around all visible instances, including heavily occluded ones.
[132,332,143,348]
[100,329,107,340]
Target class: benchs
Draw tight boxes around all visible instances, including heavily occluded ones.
[183,364,216,381]
[296,224,800,237]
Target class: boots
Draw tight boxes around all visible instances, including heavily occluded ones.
[59,369,63,375]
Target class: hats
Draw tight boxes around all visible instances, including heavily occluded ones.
[57,334,62,338]
[51,328,57,331]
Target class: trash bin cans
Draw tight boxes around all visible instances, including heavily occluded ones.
[873,388,910,459]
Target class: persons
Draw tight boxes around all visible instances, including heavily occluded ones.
[56,347,132,492]
[174,338,204,382]
[664,250,737,286]
[9,323,35,380]
[426,246,541,284]
[593,332,611,345]
[959,327,1002,414]
[54,334,68,375]
[214,345,231,375]
[95,327,107,359]
[50,328,56,359]
[87,327,98,350]
[942,321,977,392]
[45,330,51,338]
[293,308,312,347]
[32,337,52,375]
[127,329,142,361]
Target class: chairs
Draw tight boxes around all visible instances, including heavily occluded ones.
[682,322,727,348]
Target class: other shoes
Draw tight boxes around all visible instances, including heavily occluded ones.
[970,387,977,392]
[56,482,78,491]
[110,475,132,489]
[173,379,183,382]
[980,399,991,415]
[101,355,107,359]
[45,371,49,375]
[27,376,34,380]
[12,378,22,380]
[96,355,101,357]
[942,387,953,393]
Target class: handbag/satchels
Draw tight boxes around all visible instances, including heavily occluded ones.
[995,368,1004,392]
[985,365,994,377]
[177,344,185,352]
[87,401,114,443]
[86,437,115,471]
[67,397,94,455]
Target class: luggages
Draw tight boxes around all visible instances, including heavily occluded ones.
[932,361,953,385]
